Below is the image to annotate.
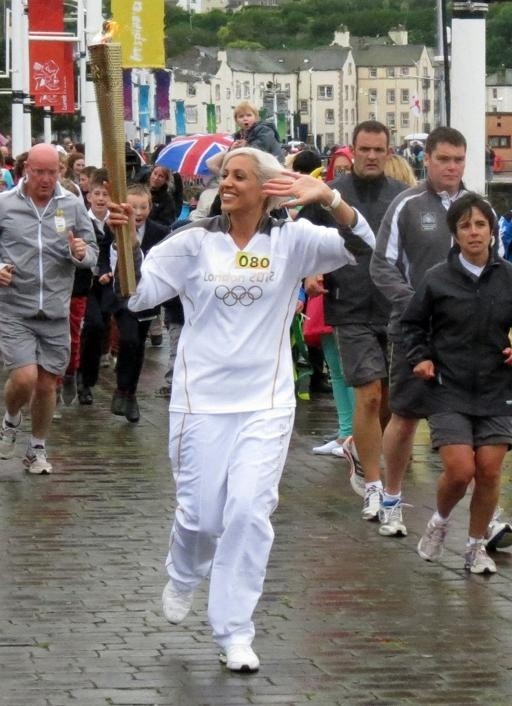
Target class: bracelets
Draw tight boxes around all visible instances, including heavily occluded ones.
[319,186,341,211]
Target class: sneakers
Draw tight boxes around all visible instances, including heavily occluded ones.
[219,641,260,674]
[164,581,195,624]
[314,437,512,575]
[1,372,140,474]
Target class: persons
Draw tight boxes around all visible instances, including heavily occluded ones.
[1,129,206,475]
[497,211,511,260]
[396,192,512,578]
[292,119,416,523]
[489,146,495,182]
[284,134,427,498]
[105,142,382,676]
[227,102,286,163]
[369,128,512,556]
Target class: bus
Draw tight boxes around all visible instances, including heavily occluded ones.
[404,132,429,149]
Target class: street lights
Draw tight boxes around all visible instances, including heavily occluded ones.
[297,58,310,141]
[271,58,284,134]
